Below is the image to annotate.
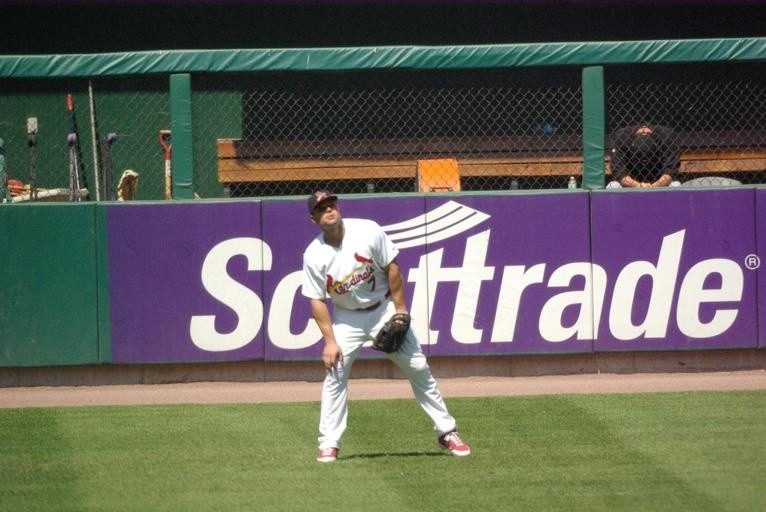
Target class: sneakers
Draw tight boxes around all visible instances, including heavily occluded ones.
[438,431,471,456]
[317,448,338,462]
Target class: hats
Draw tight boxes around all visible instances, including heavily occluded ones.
[309,190,338,212]
[633,134,658,162]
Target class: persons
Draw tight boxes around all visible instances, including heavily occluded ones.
[300,189,471,462]
[607,123,683,188]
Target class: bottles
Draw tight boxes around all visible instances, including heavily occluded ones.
[568,176,577,189]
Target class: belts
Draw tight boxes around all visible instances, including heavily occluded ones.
[356,290,391,311]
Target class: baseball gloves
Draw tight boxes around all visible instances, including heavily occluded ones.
[372,313,411,354]
